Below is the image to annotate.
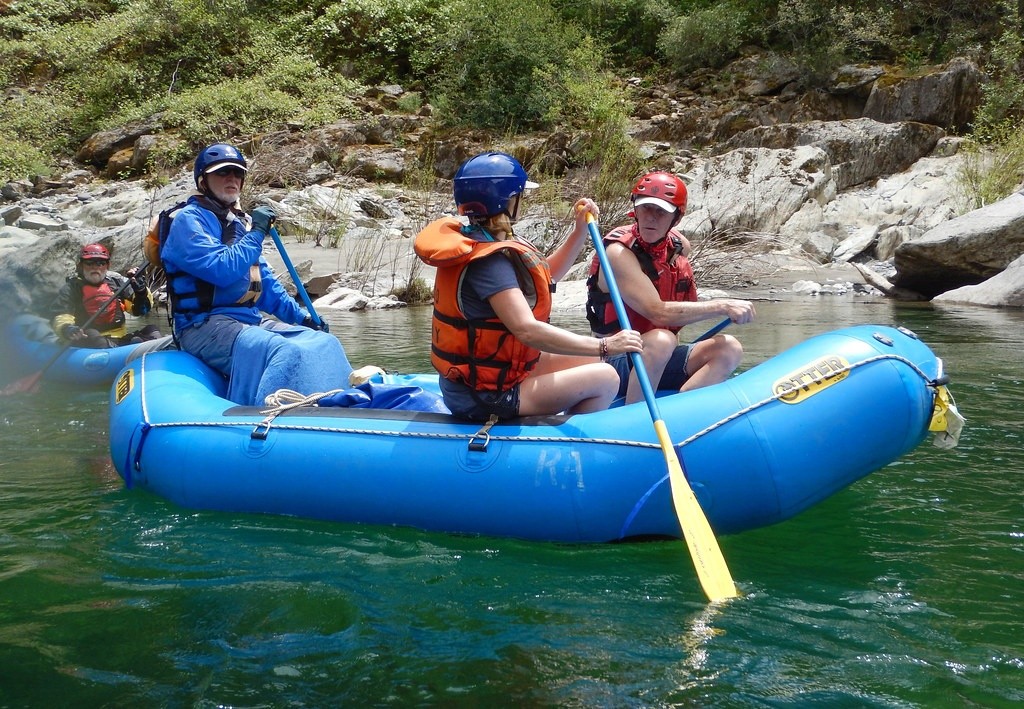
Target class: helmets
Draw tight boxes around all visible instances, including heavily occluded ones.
[194,144,247,190]
[80,242,110,263]
[453,153,529,216]
[632,172,688,217]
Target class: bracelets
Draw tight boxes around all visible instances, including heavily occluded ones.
[599,337,608,361]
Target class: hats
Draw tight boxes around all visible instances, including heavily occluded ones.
[523,180,538,189]
[634,194,677,213]
[205,161,246,174]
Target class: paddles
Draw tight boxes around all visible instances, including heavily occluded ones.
[691,317,736,344]
[579,202,742,603]
[4,260,149,398]
[266,221,319,323]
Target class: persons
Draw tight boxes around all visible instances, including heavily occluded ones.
[48,242,163,349]
[160,143,355,407]
[431,151,645,420]
[585,171,757,405]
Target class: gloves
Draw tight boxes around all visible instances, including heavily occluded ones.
[252,206,277,234]
[303,313,329,333]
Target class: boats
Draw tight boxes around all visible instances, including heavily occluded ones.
[6,310,176,387]
[108,324,946,547]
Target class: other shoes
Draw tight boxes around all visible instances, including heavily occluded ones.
[130,336,143,344]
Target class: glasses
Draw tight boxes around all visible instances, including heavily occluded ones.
[82,259,107,266]
[213,167,244,177]
